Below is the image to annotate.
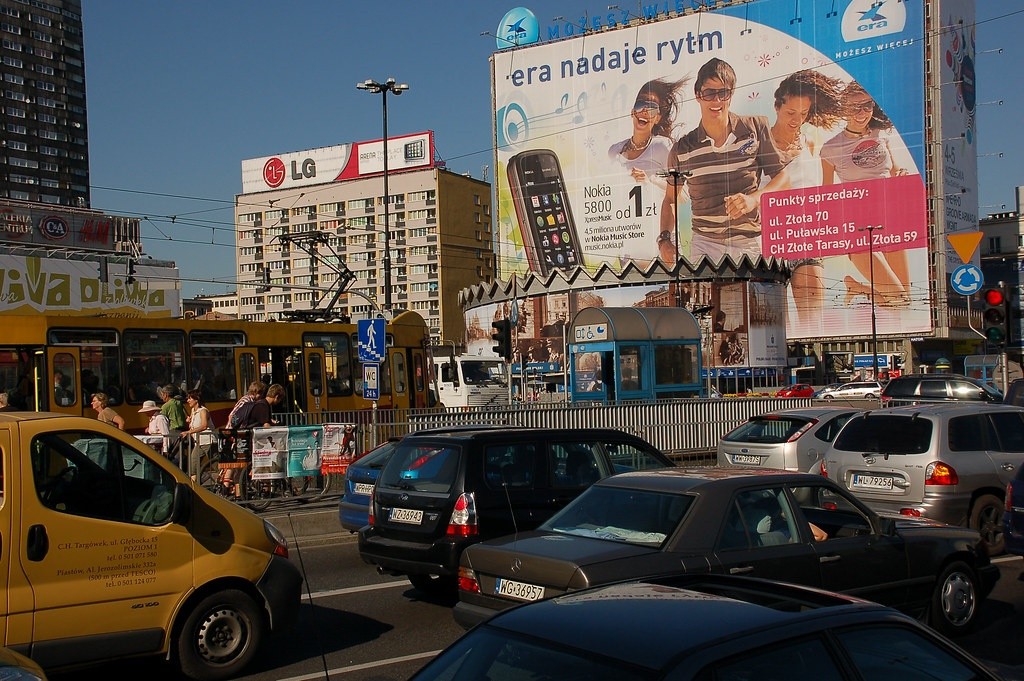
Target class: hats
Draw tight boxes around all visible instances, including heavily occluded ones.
[138,401,162,412]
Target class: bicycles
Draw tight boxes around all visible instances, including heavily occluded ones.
[164,433,332,512]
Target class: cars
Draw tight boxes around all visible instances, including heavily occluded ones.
[716,407,866,508]
[451,466,1000,640]
[1003,378,1024,408]
[819,404,1024,556]
[775,381,884,402]
[0,646,47,681]
[356,425,679,602]
[1002,460,1024,558]
[878,375,1003,408]
[338,437,403,565]
[406,574,1024,681]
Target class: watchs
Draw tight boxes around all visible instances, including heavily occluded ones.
[657,230,671,243]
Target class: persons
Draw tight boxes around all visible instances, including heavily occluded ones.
[607,74,693,267]
[657,57,910,336]
[713,310,746,365]
[757,498,828,544]
[255,424,355,471]
[0,358,301,499]
[510,338,567,369]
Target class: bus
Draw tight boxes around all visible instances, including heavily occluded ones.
[0,310,458,473]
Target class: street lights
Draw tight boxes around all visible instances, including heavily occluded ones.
[858,224,883,381]
[656,170,692,307]
[355,77,409,311]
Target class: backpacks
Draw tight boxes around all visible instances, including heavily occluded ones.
[231,400,269,430]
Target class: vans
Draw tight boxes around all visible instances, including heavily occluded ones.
[0,411,302,681]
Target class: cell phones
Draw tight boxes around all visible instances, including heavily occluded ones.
[508,149,586,277]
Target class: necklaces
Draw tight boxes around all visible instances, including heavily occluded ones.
[628,136,651,151]
[844,127,868,136]
[774,130,799,150]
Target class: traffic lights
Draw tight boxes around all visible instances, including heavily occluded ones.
[492,320,510,357]
[979,285,1007,346]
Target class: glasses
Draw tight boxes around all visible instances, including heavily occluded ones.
[635,100,660,115]
[846,101,876,115]
[697,88,733,102]
